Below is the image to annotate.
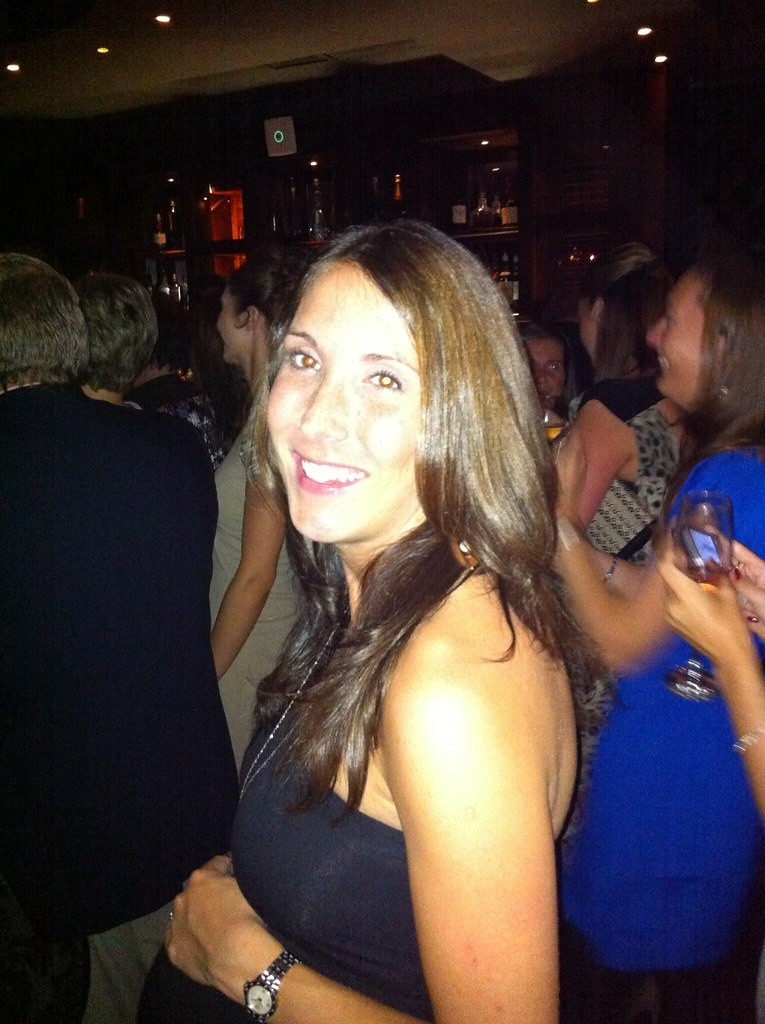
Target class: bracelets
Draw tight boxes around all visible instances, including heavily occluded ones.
[731,726,764,752]
[598,555,617,583]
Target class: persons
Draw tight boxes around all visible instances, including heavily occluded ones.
[549,243,765,1024]
[653,515,765,820]
[0,251,242,1024]
[135,218,615,1024]
[73,230,689,777]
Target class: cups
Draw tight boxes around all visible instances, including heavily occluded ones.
[541,395,567,444]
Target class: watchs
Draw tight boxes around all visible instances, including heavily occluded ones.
[243,951,301,1024]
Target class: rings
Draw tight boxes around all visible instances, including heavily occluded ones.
[169,912,173,920]
[736,561,741,569]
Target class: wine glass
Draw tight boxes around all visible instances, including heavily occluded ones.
[662,491,732,700]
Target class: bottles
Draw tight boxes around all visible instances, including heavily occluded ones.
[366,173,408,226]
[451,187,517,227]
[154,200,182,249]
[159,269,188,309]
[288,178,337,242]
[500,250,519,307]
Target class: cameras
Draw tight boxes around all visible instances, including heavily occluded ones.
[681,527,727,572]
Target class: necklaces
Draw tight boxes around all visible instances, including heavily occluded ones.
[237,606,347,805]
